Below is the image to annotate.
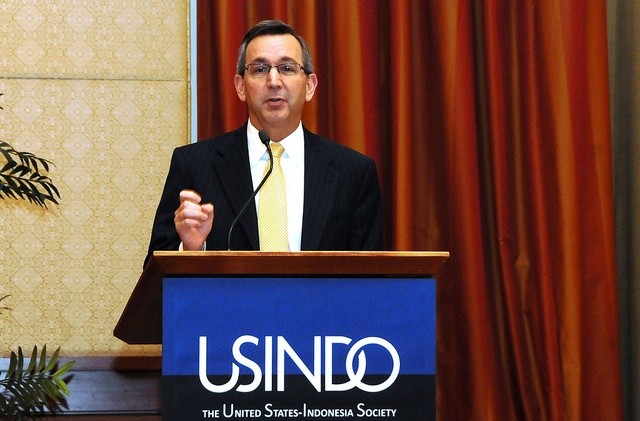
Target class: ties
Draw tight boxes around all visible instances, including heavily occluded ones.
[258,142,290,251]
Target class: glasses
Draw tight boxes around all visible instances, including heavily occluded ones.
[240,62,310,77]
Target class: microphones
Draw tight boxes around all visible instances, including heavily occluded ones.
[258,130,270,143]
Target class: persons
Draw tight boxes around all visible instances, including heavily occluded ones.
[143,19,386,270]
